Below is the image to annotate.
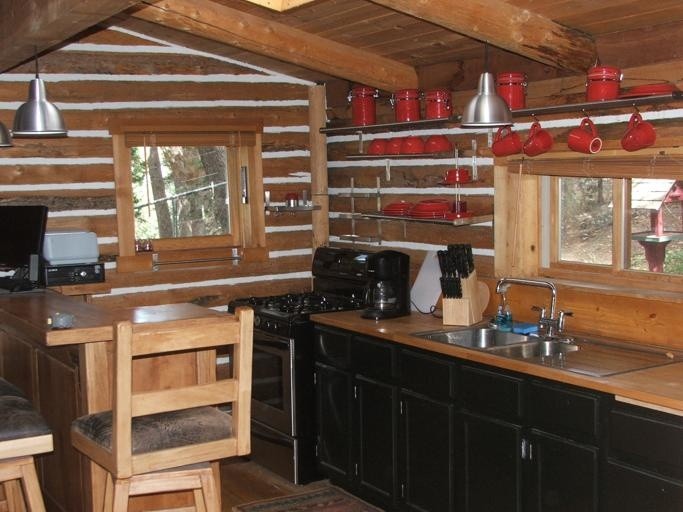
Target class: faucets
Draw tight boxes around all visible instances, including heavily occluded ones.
[496,278,557,318]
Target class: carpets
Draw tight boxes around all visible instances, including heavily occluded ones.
[222,479,385,511]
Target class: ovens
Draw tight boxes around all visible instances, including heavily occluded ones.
[248,329,330,483]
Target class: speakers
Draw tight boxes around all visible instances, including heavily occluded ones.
[28,255,39,287]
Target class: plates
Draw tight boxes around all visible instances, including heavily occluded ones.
[382,199,452,219]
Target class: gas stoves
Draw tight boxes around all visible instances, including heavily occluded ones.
[227,288,360,335]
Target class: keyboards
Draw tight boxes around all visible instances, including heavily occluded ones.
[0,277,15,289]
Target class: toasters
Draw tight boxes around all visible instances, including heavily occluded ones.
[42,229,105,286]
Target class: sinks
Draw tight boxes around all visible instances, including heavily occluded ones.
[484,340,580,358]
[407,322,549,350]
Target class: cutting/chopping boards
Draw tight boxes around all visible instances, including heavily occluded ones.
[405,248,441,312]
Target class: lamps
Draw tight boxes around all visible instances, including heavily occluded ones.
[0,116,11,149]
[7,43,69,135]
[460,39,513,127]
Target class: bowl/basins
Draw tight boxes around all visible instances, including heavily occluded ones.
[443,169,471,183]
[367,135,452,155]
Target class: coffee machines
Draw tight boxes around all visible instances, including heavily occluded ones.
[358,249,411,321]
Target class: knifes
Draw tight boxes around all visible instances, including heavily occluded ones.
[433,242,473,298]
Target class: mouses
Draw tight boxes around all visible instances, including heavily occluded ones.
[10,283,22,292]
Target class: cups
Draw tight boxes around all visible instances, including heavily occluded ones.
[284,198,299,208]
[619,111,656,152]
[567,119,602,154]
[521,121,553,157]
[490,124,522,160]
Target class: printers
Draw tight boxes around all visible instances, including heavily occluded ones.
[42,229,99,265]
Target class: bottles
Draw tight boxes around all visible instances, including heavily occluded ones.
[493,304,511,327]
[135,234,152,251]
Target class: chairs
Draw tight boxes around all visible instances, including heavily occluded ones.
[70,306,254,511]
[0,375,55,510]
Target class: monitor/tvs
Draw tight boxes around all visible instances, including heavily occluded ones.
[0,205,49,265]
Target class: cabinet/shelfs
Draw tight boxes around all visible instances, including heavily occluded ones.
[520,336,678,511]
[338,113,492,228]
[401,310,522,510]
[312,305,398,511]
[606,405,682,510]
